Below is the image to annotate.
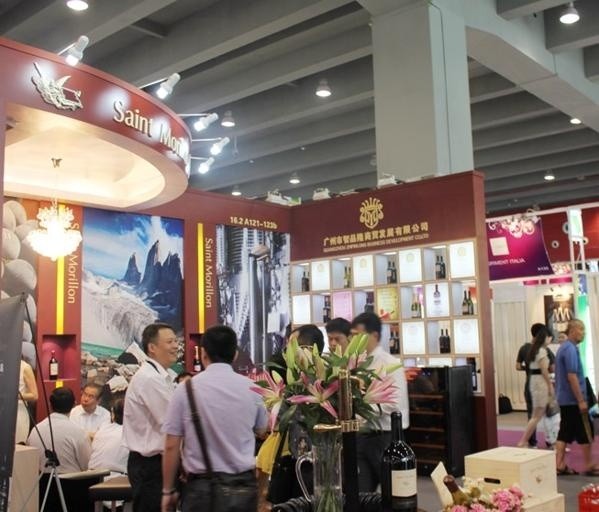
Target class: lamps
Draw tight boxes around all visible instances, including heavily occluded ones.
[54,34,92,65]
[25,155,83,263]
[287,171,301,184]
[191,156,215,173]
[231,185,241,195]
[558,0,580,23]
[314,73,333,98]
[139,71,181,101]
[190,137,230,155]
[543,170,555,181]
[179,111,219,130]
[221,110,236,128]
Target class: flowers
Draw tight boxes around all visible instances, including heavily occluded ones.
[246,330,407,511]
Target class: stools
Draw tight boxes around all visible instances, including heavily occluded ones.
[88,475,135,511]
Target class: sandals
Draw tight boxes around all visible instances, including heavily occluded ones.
[556,463,580,475]
[586,467,598,476]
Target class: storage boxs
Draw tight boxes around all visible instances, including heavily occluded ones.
[519,494,566,512]
[463,446,559,503]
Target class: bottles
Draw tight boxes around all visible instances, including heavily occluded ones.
[383,255,474,354]
[379,408,417,512]
[471,367,478,391]
[443,475,472,509]
[48,349,59,380]
[192,345,202,373]
[300,265,375,324]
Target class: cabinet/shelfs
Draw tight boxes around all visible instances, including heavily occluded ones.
[290,235,489,399]
[407,364,474,478]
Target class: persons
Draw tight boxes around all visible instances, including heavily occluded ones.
[553,318,598,476]
[24,386,89,474]
[351,310,411,493]
[288,323,325,359]
[159,324,273,512]
[558,330,569,345]
[86,396,130,511]
[324,316,354,353]
[175,372,193,383]
[122,322,182,511]
[69,383,113,457]
[16,352,39,446]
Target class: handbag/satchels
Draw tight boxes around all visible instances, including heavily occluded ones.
[498,392,513,414]
[546,398,561,417]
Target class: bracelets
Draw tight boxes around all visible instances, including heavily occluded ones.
[161,488,178,494]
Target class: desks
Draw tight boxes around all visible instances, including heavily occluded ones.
[8,444,42,512]
